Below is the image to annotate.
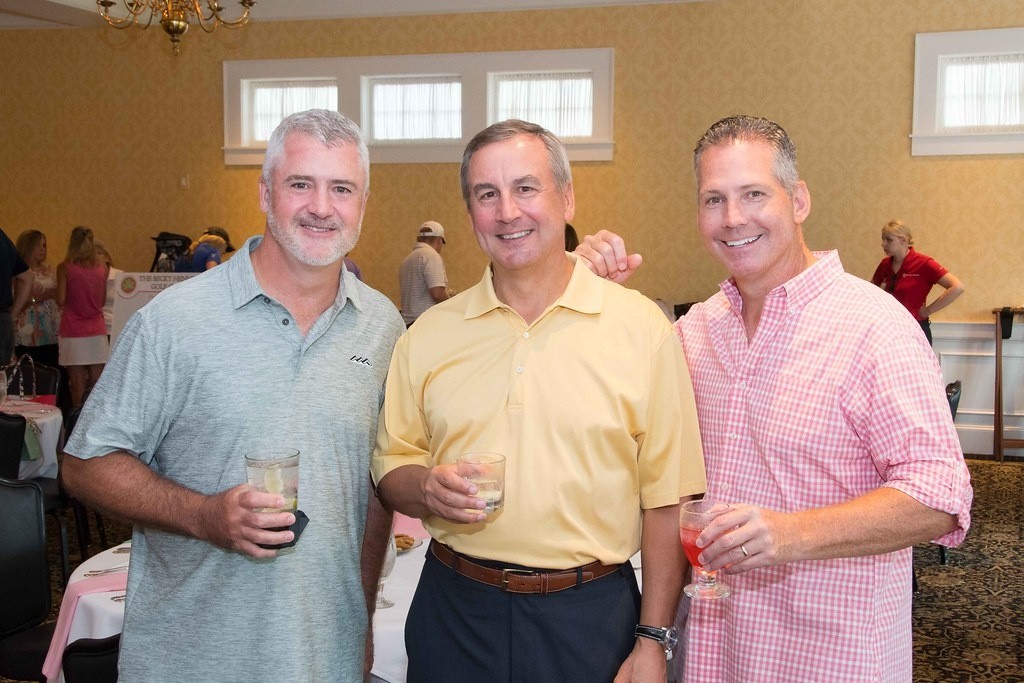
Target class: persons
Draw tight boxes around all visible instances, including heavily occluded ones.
[389,214,455,326]
[869,213,967,350]
[0,221,236,414]
[575,116,973,683]
[370,117,707,683]
[58,109,407,682]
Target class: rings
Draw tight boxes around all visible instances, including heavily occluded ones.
[741,546,747,555]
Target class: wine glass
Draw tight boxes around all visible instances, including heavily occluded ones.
[679,499,733,600]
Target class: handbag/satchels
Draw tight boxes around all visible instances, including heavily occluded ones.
[2,354,57,407]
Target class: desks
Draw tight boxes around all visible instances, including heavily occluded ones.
[0,402,63,477]
[44,520,644,683]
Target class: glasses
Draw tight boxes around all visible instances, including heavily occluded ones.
[889,274,896,291]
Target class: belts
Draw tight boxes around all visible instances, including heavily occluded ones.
[430,540,626,596]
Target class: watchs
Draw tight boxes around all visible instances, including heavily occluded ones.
[634,625,678,653]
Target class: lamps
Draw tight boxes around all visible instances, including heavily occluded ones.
[96,0,256,58]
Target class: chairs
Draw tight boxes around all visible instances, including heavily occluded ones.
[46,494,111,585]
[0,412,26,480]
[0,478,59,681]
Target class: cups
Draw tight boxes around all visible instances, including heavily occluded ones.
[244,447,301,515]
[457,452,507,513]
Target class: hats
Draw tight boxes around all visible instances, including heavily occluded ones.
[203,226,235,252]
[417,221,447,245]
